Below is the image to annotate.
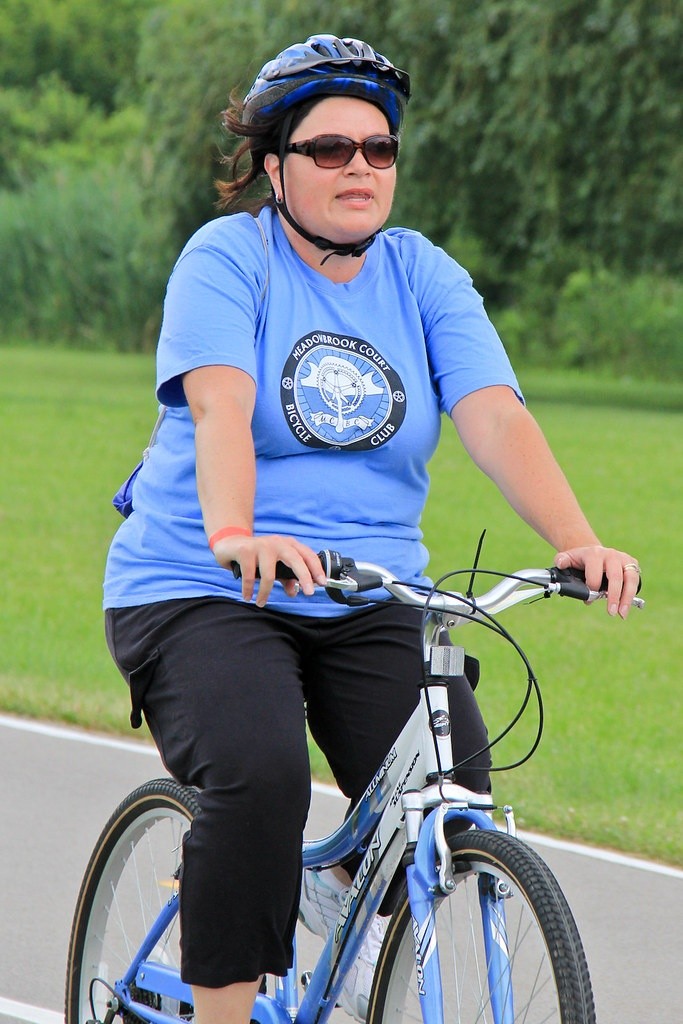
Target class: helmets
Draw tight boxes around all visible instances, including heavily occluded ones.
[241,32,412,134]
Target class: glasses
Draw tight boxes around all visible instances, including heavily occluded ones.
[284,134,400,170]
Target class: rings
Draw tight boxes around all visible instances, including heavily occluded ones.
[624,563,641,575]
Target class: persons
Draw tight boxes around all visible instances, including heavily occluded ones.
[98,33,641,1022]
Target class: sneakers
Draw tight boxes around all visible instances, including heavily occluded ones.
[296,869,394,1023]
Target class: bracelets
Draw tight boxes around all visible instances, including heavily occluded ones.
[208,525,254,551]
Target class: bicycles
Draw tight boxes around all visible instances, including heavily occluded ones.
[66,545,647,1023]
